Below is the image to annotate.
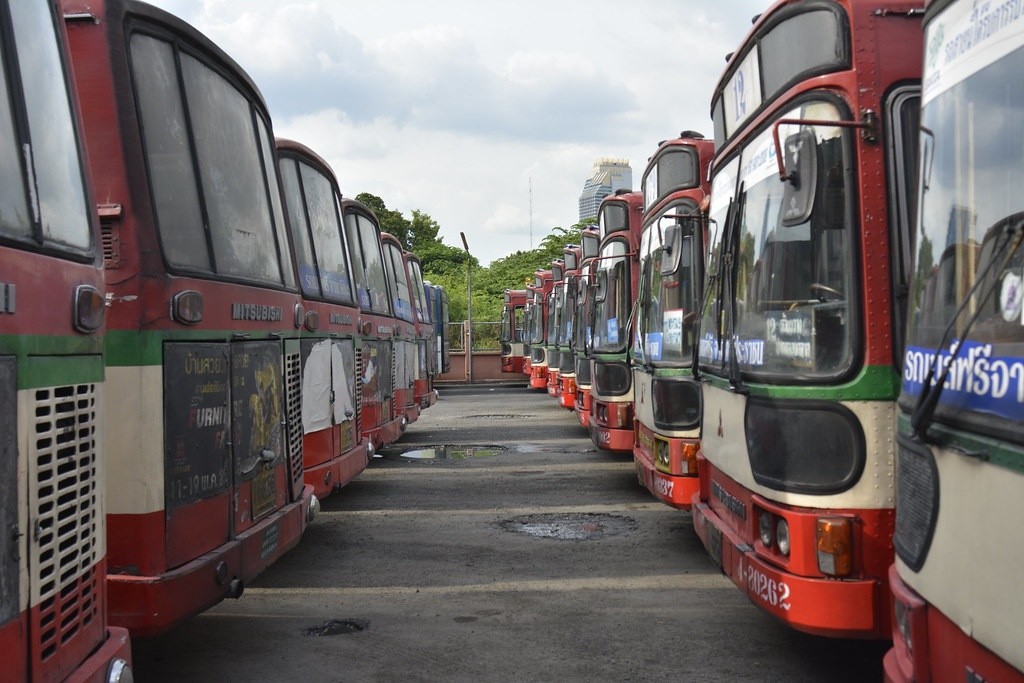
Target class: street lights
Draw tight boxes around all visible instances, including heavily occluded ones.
[919,124,935,237]
[460,232,472,382]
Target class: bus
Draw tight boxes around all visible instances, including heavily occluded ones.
[273,137,376,501]
[631,130,714,511]
[500,225,600,428]
[584,188,643,451]
[404,251,439,409]
[381,232,421,424]
[341,197,408,456]
[693,0,1024,640]
[882,0,1024,681]
[1,0,133,683]
[61,0,321,636]
